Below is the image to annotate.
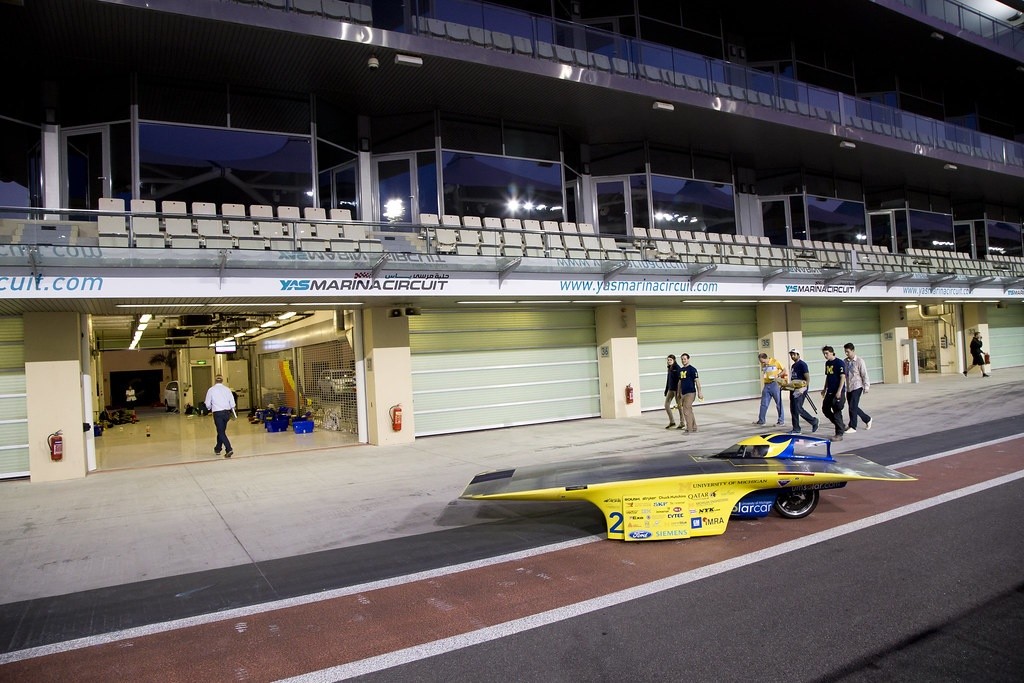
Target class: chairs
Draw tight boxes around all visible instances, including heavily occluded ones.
[97,196,1024,278]
[237,0,1024,168]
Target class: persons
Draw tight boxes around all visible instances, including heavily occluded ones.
[789,348,820,435]
[204,375,236,458]
[822,346,847,442]
[752,353,786,427]
[664,353,703,433]
[962,332,990,377]
[126,384,137,410]
[844,343,873,433]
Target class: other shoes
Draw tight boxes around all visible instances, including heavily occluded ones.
[215,449,222,454]
[225,451,233,457]
[774,423,785,427]
[785,431,801,435]
[844,428,856,433]
[866,418,872,429]
[812,418,820,432]
[752,423,766,426]
[665,423,675,428]
[830,436,843,441]
[677,426,685,430]
[983,373,989,377]
[963,371,967,377]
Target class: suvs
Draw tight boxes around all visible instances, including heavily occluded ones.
[318,369,356,404]
[164,380,178,412]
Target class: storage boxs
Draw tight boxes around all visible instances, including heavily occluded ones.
[292,421,315,434]
[264,420,289,433]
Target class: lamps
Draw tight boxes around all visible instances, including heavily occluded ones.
[394,54,423,68]
[997,303,1008,308]
[653,102,675,112]
[944,163,958,171]
[930,32,945,41]
[839,140,856,149]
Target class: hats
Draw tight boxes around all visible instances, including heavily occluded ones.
[216,374,223,380]
[789,348,799,353]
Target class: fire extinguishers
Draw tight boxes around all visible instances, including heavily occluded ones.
[626,383,634,403]
[903,359,910,374]
[47,429,63,460]
[389,403,402,430]
[985,353,990,364]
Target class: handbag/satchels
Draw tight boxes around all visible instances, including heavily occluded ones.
[230,408,237,421]
